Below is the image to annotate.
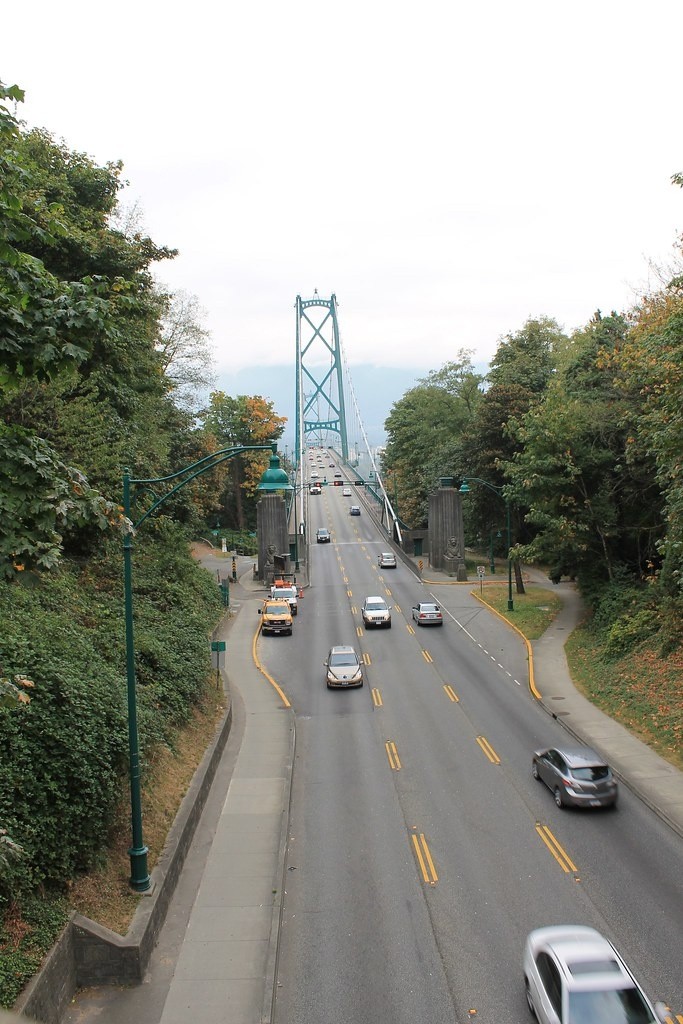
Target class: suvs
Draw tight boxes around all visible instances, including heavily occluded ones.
[267,588,298,615]
[257,600,296,636]
[360,597,391,629]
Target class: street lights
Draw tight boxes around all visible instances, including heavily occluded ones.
[458,474,516,613]
[121,443,294,893]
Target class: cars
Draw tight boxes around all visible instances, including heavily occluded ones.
[323,645,364,689]
[411,602,443,627]
[311,470,318,478]
[335,470,341,477]
[531,745,619,811]
[315,528,330,543]
[342,488,351,496]
[378,552,396,568]
[309,449,330,462]
[311,462,316,467]
[348,506,360,516]
[521,924,662,1024]
[319,463,325,468]
[310,487,321,495]
[329,462,335,467]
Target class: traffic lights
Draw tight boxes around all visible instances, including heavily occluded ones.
[354,480,364,487]
[334,481,343,487]
[315,482,328,487]
